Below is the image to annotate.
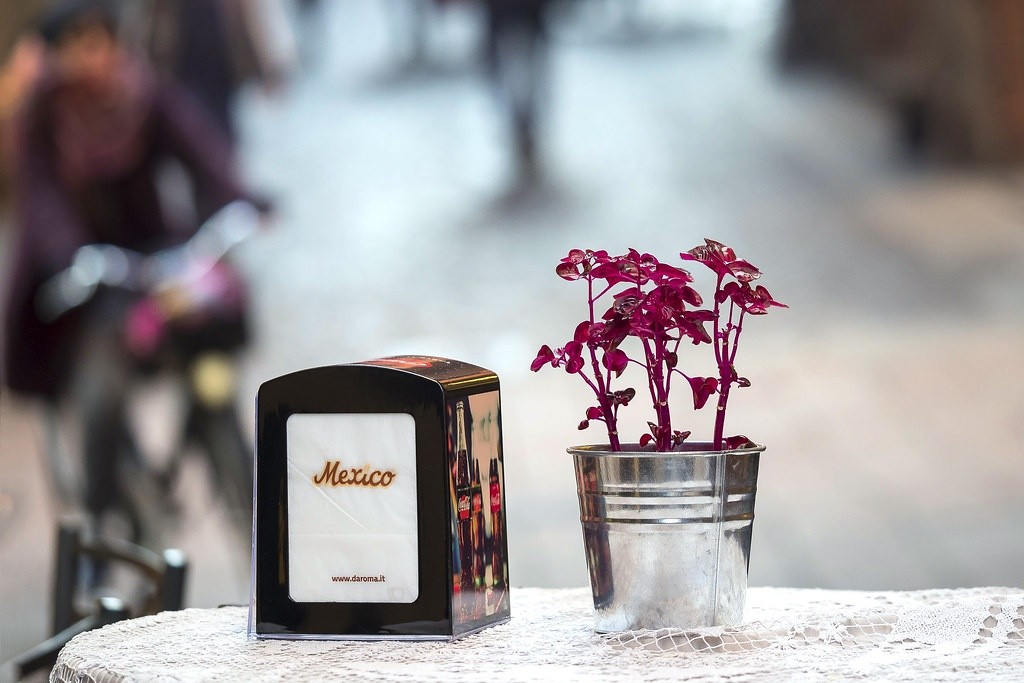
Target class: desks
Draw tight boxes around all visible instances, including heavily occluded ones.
[48,586,1024,680]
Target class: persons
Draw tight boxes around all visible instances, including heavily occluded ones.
[0,1,275,548]
[482,1,568,205]
[111,0,289,210]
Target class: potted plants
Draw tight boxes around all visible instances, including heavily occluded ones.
[533,238,790,630]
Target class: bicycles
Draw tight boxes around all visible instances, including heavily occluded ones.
[41,202,253,578]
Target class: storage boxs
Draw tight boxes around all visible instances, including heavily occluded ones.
[251,355,512,642]
